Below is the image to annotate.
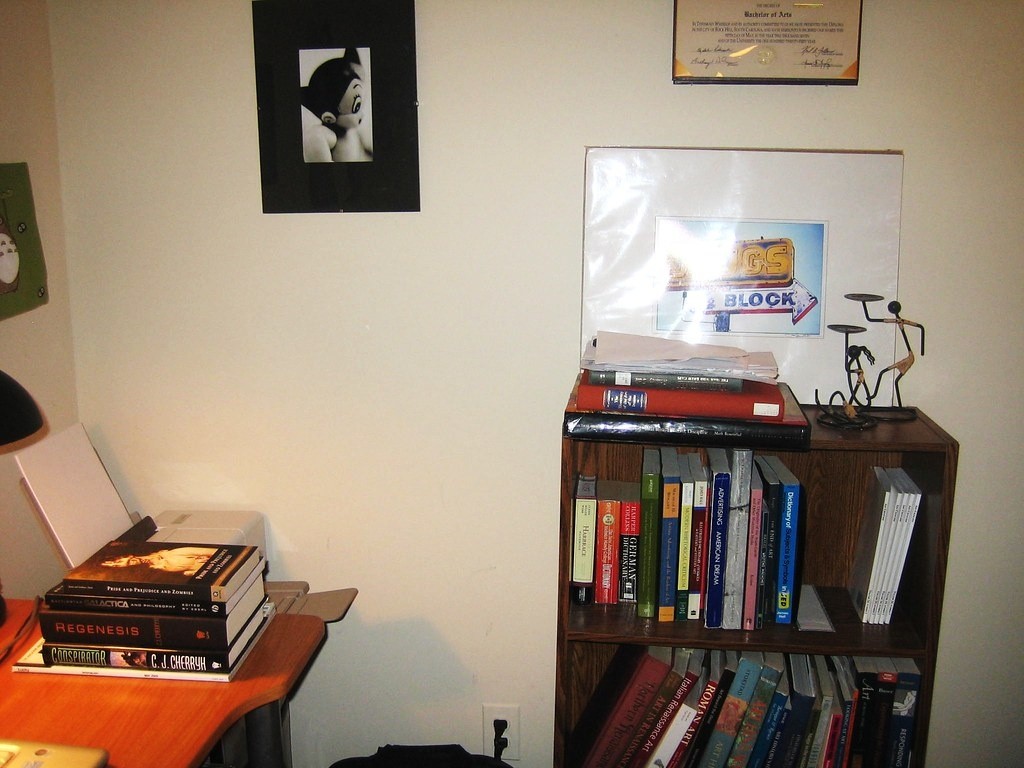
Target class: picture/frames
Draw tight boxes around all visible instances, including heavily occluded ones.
[252,1,425,212]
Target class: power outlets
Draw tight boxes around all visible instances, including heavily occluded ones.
[482,703,521,760]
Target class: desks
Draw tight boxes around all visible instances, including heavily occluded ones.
[0,600,329,768]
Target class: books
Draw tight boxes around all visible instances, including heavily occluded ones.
[560,645,926,768]
[564,330,923,633]
[9,539,277,682]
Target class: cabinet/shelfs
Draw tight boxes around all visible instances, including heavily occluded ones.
[552,372,961,768]
